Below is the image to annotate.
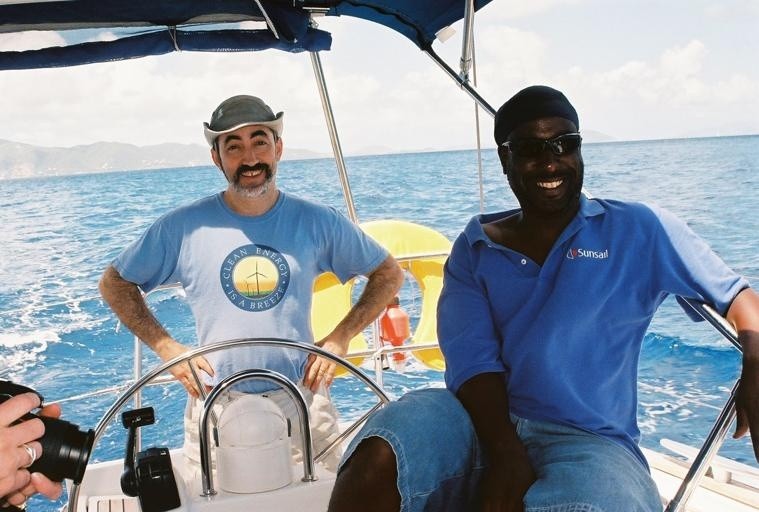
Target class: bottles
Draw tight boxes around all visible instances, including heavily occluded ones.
[379,296,411,362]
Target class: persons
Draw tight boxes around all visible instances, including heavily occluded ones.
[98,95,403,477]
[0,392,64,512]
[326,85,758,512]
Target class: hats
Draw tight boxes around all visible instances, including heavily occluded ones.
[494,86,579,146]
[203,95,284,148]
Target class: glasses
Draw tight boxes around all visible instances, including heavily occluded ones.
[501,133,583,158]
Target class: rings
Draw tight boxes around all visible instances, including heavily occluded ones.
[22,443,37,468]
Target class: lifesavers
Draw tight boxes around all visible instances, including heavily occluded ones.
[310,219,455,379]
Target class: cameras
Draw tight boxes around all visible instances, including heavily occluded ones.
[0,380,97,487]
